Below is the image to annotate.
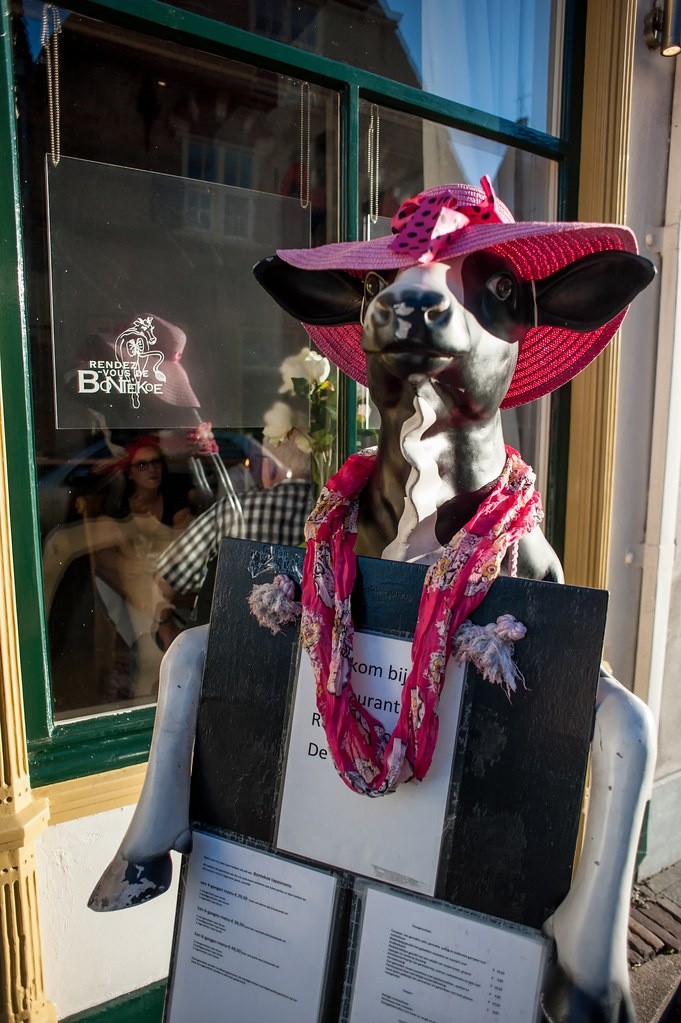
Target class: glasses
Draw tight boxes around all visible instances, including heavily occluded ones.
[253,452,269,462]
[129,457,164,471]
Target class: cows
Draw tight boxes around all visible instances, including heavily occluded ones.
[86,249,661,1023]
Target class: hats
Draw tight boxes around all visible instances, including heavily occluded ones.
[277,176,637,409]
[87,311,204,407]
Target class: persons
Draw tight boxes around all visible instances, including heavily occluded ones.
[45,388,313,700]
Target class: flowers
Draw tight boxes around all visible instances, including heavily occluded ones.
[263,345,380,494]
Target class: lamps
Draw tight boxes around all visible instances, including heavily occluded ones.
[643,0,681,57]
